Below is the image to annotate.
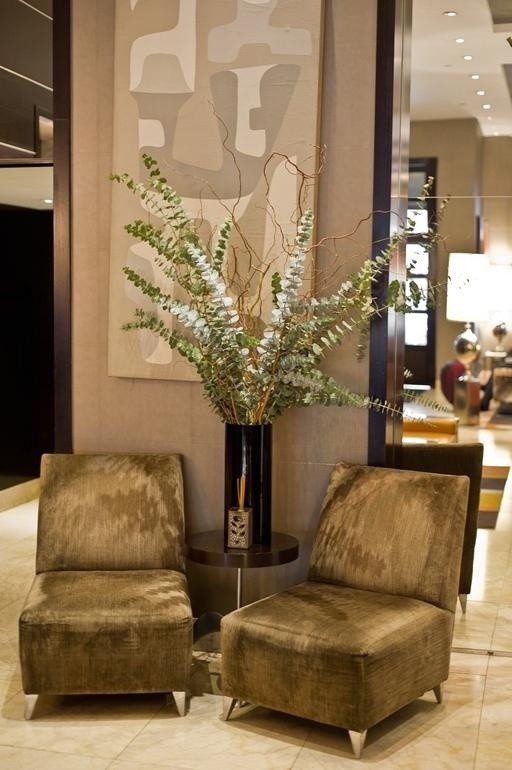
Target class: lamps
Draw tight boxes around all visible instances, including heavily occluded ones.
[444,252,496,353]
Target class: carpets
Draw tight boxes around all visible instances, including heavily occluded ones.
[472,466,511,529]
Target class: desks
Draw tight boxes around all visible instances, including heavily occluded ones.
[188,529,300,697]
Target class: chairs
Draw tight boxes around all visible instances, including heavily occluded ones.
[216,460,472,760]
[18,453,192,722]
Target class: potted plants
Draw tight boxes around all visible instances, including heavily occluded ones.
[110,99,456,549]
